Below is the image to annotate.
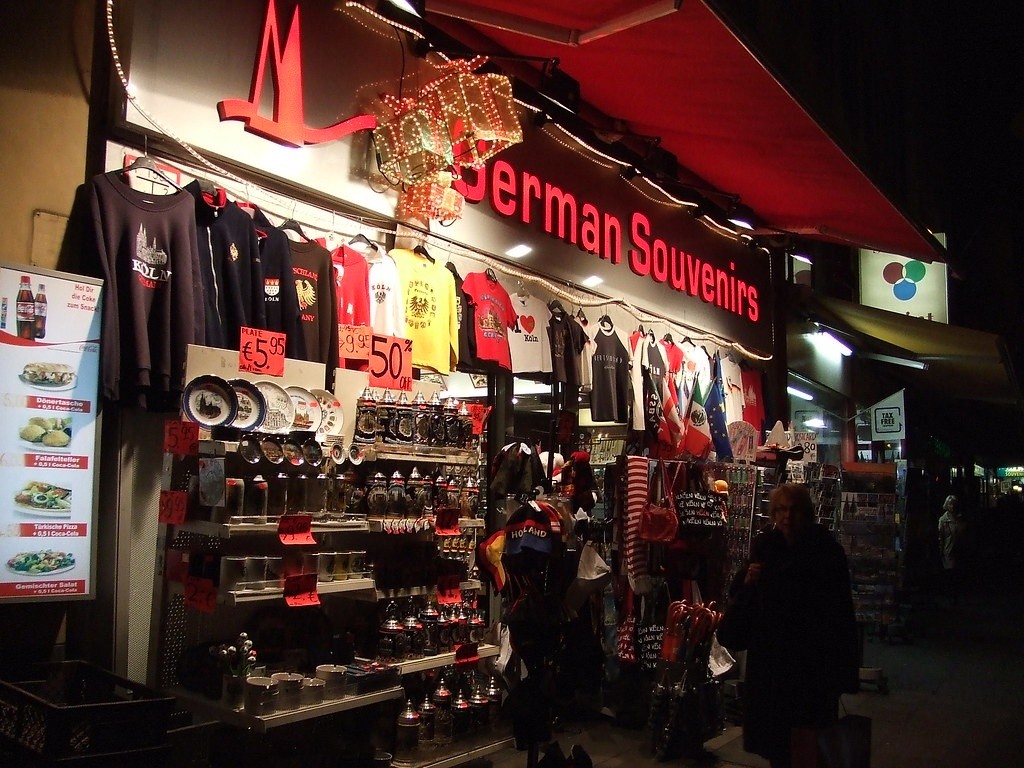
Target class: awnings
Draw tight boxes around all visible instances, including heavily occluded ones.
[815,298,1024,407]
[368,0,967,282]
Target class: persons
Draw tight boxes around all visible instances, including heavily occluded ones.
[716,485,858,768]
[938,499,976,557]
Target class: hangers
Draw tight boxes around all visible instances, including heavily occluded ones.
[517,276,530,298]
[716,343,720,358]
[278,197,311,243]
[727,343,737,363]
[114,134,184,194]
[445,245,459,279]
[740,352,752,369]
[348,216,378,251]
[649,319,655,336]
[637,314,644,335]
[414,234,435,264]
[241,183,255,220]
[663,323,674,344]
[681,331,695,347]
[549,290,565,314]
[199,166,219,200]
[600,303,612,327]
[486,258,498,283]
[325,210,343,247]
[575,300,588,322]
[701,338,711,358]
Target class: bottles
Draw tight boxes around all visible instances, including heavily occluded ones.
[14,275,47,342]
[355,387,503,763]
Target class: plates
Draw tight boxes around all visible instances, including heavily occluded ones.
[4,560,75,576]
[253,380,297,434]
[331,444,345,464]
[226,379,267,433]
[19,373,78,391]
[303,440,323,466]
[284,385,323,432]
[239,434,262,464]
[182,375,238,432]
[283,438,305,466]
[348,445,362,465]
[10,492,71,517]
[19,438,71,453]
[262,436,285,465]
[310,388,344,442]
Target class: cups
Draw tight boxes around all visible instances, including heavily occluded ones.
[244,664,347,716]
[220,552,365,594]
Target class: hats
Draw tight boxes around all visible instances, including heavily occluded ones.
[479,499,611,750]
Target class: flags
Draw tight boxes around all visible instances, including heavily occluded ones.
[643,369,733,459]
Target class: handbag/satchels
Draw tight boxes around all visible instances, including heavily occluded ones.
[790,692,873,768]
[615,458,737,758]
[717,527,774,651]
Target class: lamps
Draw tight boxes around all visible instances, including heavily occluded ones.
[620,167,756,230]
[534,112,682,183]
[817,329,852,356]
[695,208,817,265]
[388,0,427,19]
[414,38,580,115]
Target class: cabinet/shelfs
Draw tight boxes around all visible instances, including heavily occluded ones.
[168,430,912,768]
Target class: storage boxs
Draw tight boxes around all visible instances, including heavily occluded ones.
[0,659,177,768]
